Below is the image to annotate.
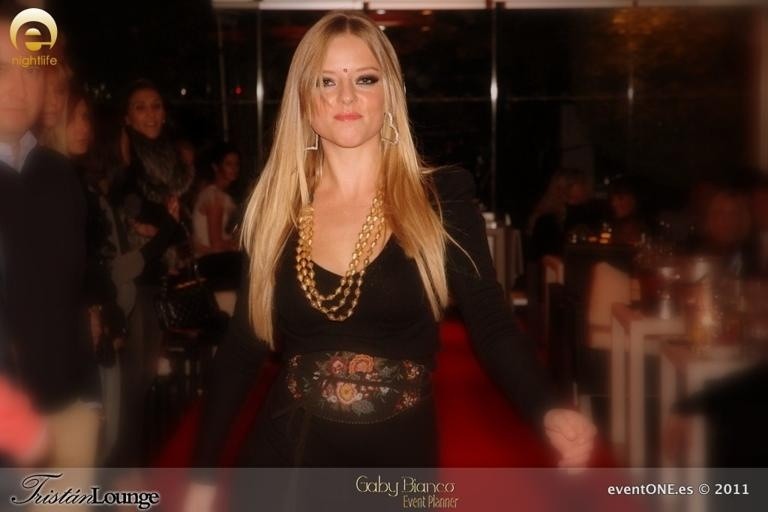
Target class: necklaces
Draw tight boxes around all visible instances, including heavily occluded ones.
[295,152,388,322]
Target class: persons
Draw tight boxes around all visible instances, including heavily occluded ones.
[1,0,194,512]
[195,145,248,256]
[523,160,766,403]
[176,12,597,512]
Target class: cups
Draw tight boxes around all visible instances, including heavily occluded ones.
[635,215,744,354]
